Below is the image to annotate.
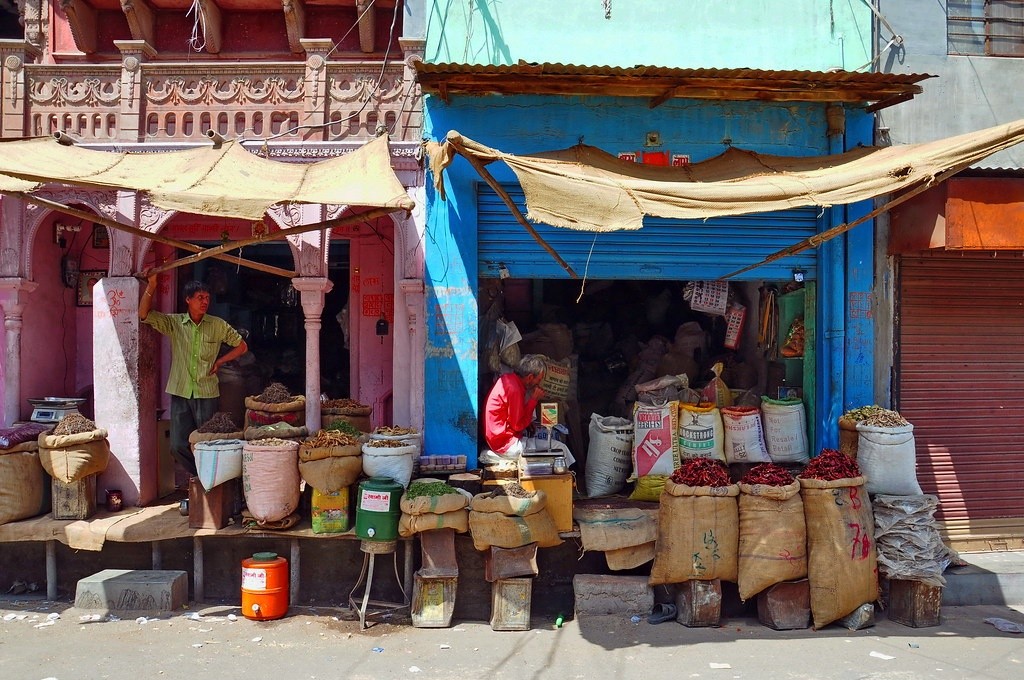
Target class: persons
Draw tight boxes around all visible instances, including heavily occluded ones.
[138,264,248,492]
[483,354,577,467]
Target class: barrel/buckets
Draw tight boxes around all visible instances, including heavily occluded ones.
[240,550,292,622]
[354,476,404,541]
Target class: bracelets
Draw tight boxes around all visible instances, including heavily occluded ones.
[145,290,152,296]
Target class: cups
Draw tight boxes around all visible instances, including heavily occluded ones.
[106,489,124,512]
[553,457,567,475]
[179,499,189,515]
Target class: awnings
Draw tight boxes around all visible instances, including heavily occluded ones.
[898,165,1023,179]
[0,138,416,280]
[403,58,943,115]
[415,119,1023,282]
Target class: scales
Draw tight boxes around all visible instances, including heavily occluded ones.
[26,395,88,423]
[518,402,566,475]
